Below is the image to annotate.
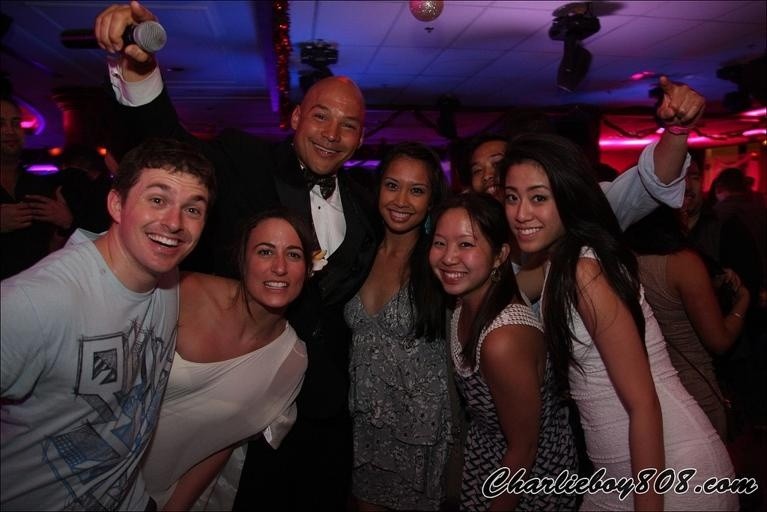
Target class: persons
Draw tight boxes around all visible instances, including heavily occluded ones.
[112,203,319,510]
[397,188,580,511]
[0,135,221,511]
[0,96,111,278]
[460,74,705,319]
[681,139,764,442]
[341,139,464,512]
[491,131,741,511]
[94,1,383,509]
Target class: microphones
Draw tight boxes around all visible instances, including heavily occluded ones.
[60,21,167,54]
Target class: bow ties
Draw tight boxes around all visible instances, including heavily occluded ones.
[293,168,336,200]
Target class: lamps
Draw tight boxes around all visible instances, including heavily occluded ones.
[548,1,600,94]
[292,38,340,95]
